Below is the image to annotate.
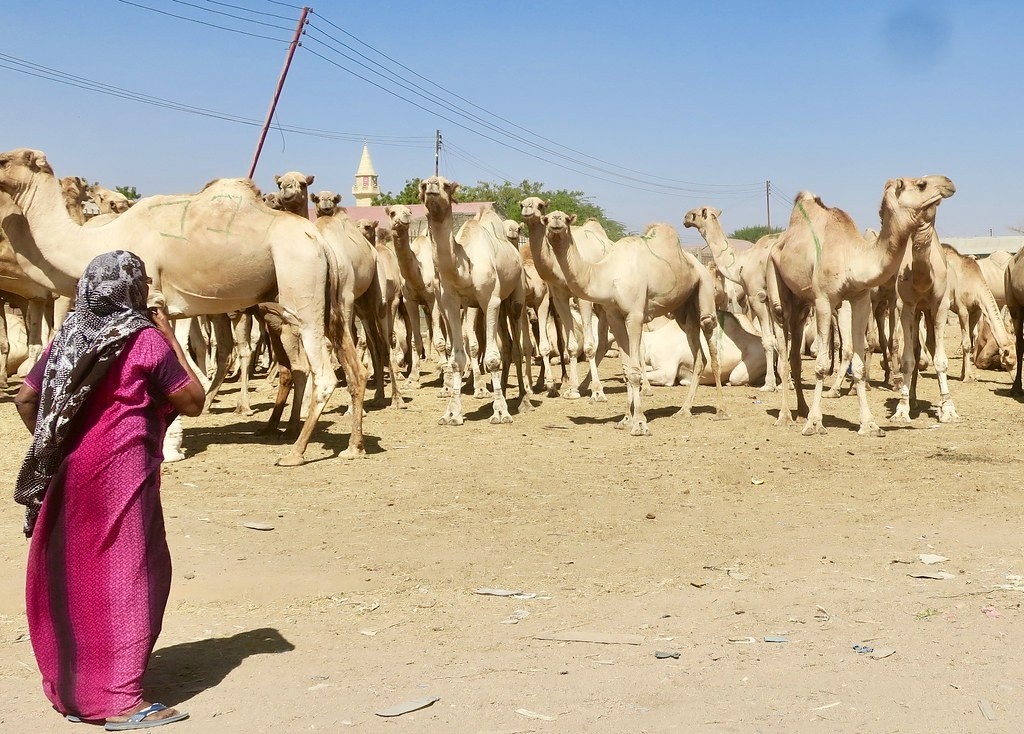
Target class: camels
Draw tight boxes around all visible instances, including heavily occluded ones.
[1,149,1024,469]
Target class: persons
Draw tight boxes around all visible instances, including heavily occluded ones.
[11,250,206,729]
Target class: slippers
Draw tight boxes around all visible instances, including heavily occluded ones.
[105,703,190,731]
